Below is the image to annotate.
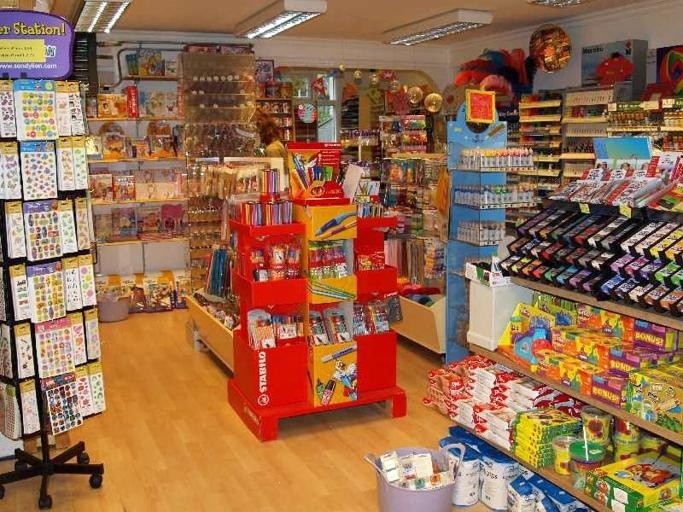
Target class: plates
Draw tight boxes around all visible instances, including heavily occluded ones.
[297,102,318,124]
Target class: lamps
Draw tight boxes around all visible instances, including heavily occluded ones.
[230,1,327,40]
[381,9,491,46]
[71,0,131,33]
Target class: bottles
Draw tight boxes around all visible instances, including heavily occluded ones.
[450,182,536,208]
[455,220,507,246]
[460,145,535,172]
